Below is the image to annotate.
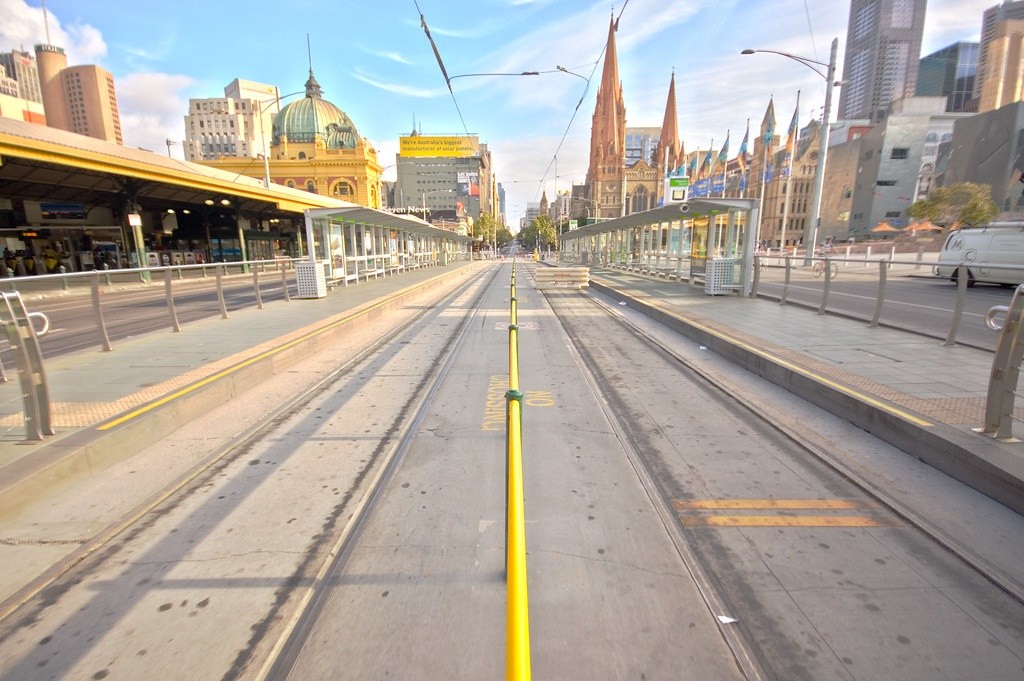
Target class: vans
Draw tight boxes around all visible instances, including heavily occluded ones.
[936,221,1024,288]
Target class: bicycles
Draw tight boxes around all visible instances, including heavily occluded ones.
[812,252,838,279]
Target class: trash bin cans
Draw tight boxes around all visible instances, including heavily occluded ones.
[542,254,546,260]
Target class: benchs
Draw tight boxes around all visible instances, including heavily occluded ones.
[361,269,387,283]
[652,265,677,280]
[429,261,433,267]
[408,263,419,271]
[388,264,405,276]
[616,263,625,270]
[326,274,348,292]
[420,261,429,269]
[689,272,705,285]
[630,262,647,273]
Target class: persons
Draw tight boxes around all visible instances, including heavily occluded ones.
[100,248,106,265]
[818,236,838,263]
[0,242,58,260]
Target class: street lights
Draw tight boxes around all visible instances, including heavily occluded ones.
[377,162,414,210]
[558,197,598,224]
[740,36,850,266]
[422,189,453,222]
[258,89,325,190]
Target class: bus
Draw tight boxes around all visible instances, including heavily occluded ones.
[17,225,128,275]
